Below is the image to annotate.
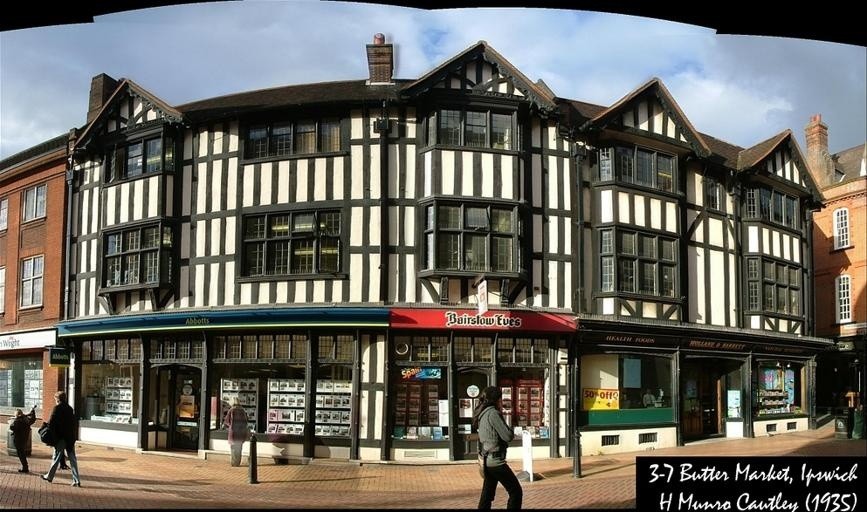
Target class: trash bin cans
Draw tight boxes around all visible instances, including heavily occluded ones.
[7,417,32,457]
[835,406,855,440]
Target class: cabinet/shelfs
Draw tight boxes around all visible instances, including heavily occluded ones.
[220,377,353,438]
[753,388,794,416]
[0,368,43,410]
[85,374,139,419]
[393,378,544,438]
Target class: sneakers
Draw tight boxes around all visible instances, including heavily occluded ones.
[40,474,52,482]
[71,483,80,487]
[18,468,29,473]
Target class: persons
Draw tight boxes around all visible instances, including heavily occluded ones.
[10,409,37,474]
[472,385,523,510]
[41,390,81,487]
[223,397,248,467]
[51,447,69,471]
[644,389,655,404]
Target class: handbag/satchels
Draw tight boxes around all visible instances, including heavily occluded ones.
[477,451,487,478]
[40,422,50,443]
